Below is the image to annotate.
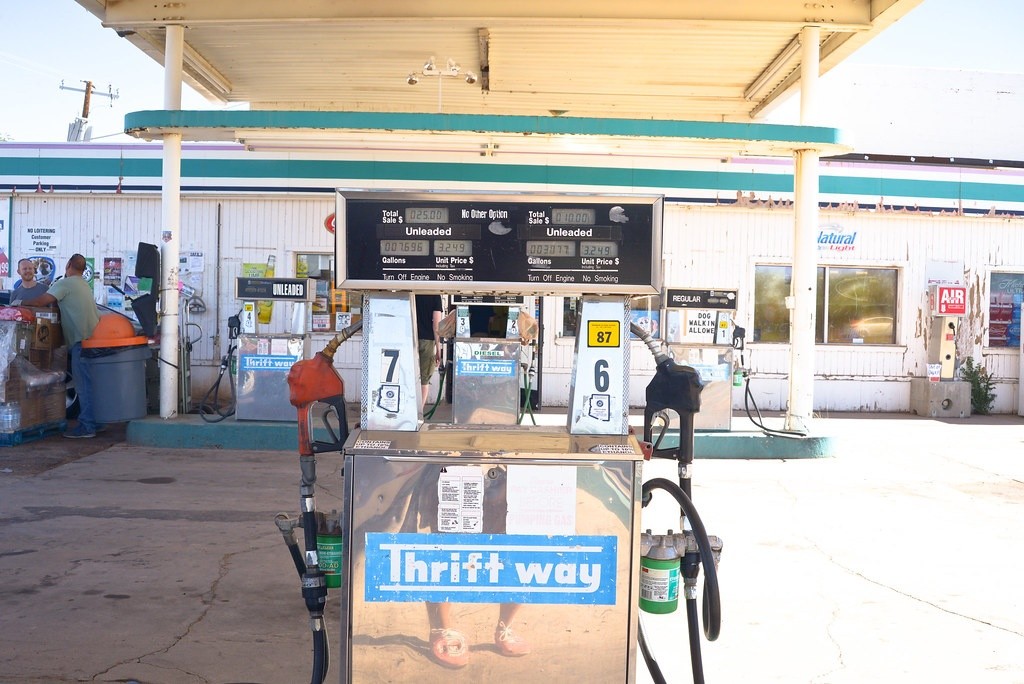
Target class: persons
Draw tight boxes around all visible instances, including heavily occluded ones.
[10,252,106,438]
[415,293,444,412]
[469,304,496,338]
[11,259,51,306]
[407,459,533,665]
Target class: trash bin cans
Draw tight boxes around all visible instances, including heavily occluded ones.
[78,312,153,424]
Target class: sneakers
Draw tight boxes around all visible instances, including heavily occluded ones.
[64,426,96,438]
[429,627,469,667]
[494,621,530,656]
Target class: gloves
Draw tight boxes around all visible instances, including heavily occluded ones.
[11,299,22,306]
